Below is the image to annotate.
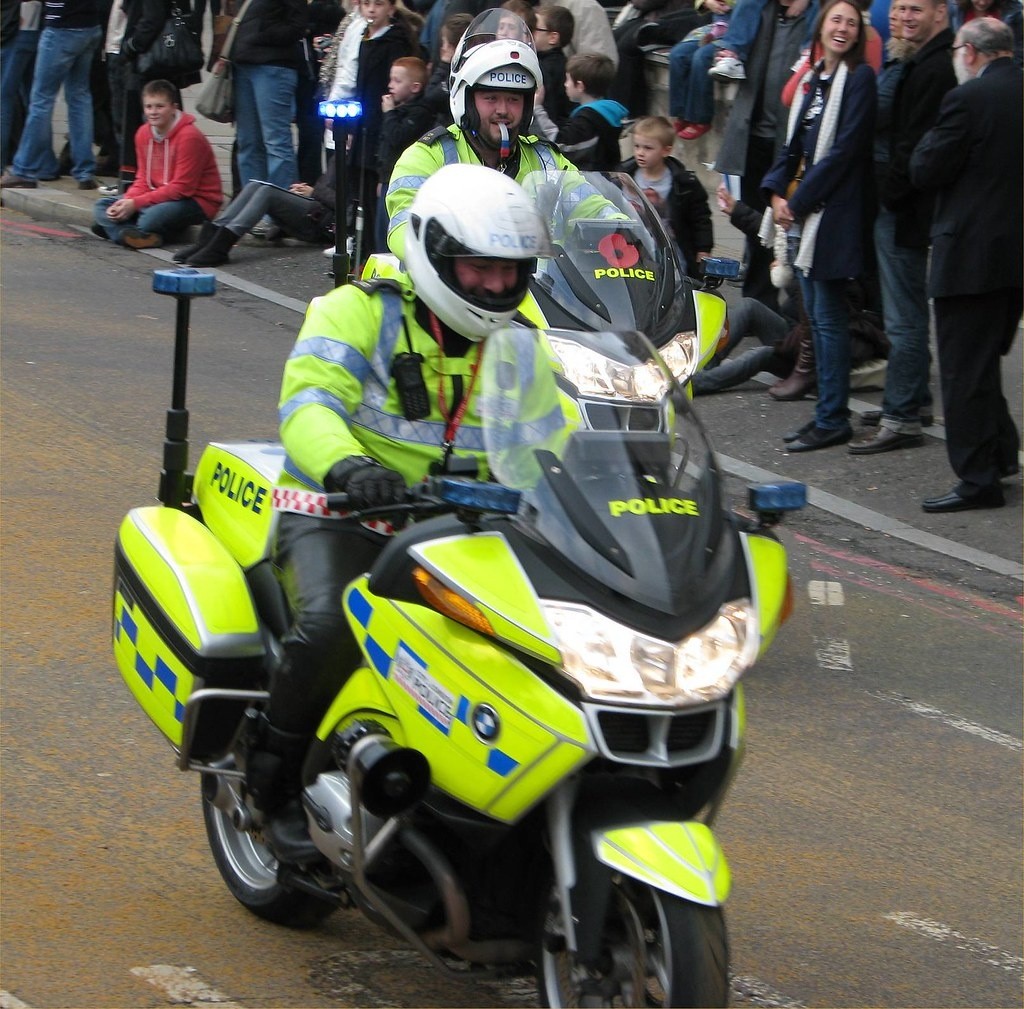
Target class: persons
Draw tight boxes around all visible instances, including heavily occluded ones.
[689,1,1024,510]
[243,165,584,868]
[1,0,739,277]
[385,37,631,346]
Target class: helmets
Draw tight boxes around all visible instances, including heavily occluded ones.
[405,163,556,345]
[448,38,542,134]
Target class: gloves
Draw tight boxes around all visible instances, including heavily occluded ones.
[324,456,408,531]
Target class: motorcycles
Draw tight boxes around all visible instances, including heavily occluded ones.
[111,255,809,1009]
[316,96,741,415]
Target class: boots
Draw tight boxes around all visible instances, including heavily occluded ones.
[769,310,815,400]
[247,628,349,806]
[172,218,219,262]
[187,225,234,267]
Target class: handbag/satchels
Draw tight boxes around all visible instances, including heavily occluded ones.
[135,15,201,88]
[194,56,235,123]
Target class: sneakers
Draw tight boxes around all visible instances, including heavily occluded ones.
[791,57,808,72]
[708,57,747,83]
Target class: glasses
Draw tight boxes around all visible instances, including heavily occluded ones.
[942,43,966,59]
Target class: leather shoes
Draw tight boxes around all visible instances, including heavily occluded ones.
[860,411,934,427]
[997,463,1019,479]
[920,491,985,513]
[847,423,925,453]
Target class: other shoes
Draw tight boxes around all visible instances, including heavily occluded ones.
[675,119,689,132]
[678,122,712,140]
[725,263,747,287]
[782,418,816,443]
[787,426,854,452]
[323,236,356,259]
[1,142,162,247]
[247,216,277,237]
